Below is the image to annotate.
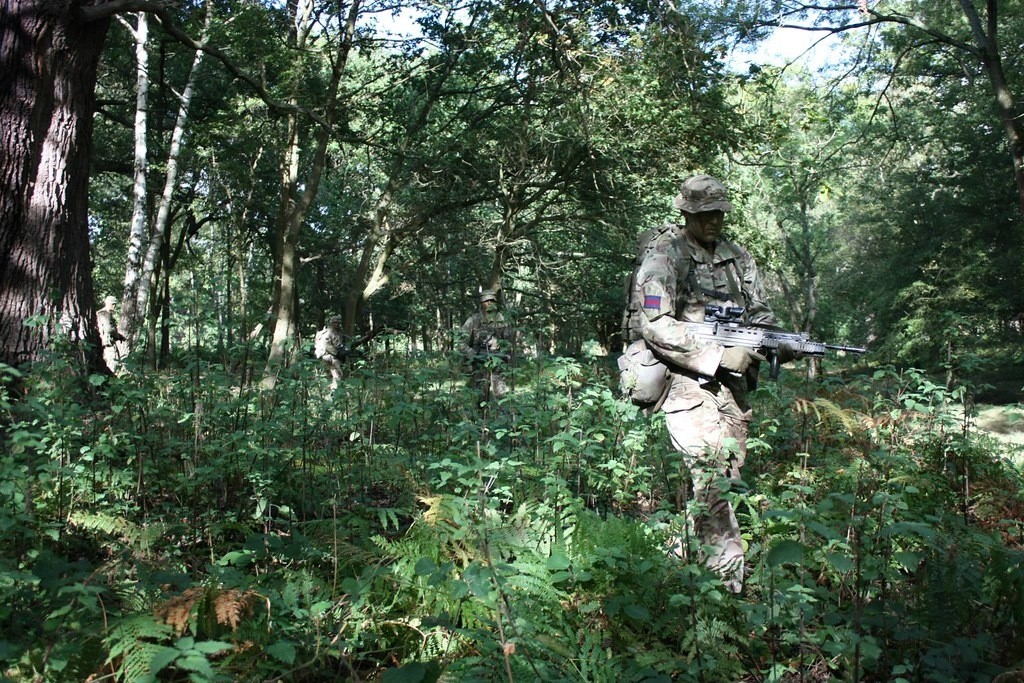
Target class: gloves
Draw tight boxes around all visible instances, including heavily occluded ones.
[719,346,767,373]
[776,342,803,365]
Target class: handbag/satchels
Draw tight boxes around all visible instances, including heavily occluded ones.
[459,358,477,374]
[617,340,670,406]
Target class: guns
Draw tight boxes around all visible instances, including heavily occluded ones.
[677,304,868,384]
[115,334,127,344]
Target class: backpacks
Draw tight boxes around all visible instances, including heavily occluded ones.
[617,222,743,353]
[314,329,333,360]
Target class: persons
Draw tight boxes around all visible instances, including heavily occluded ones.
[637,176,776,598]
[97,297,120,372]
[315,315,351,397]
[458,289,512,409]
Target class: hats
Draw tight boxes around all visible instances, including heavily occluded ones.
[673,175,731,214]
[329,316,342,323]
[104,295,118,304]
[480,288,497,303]
